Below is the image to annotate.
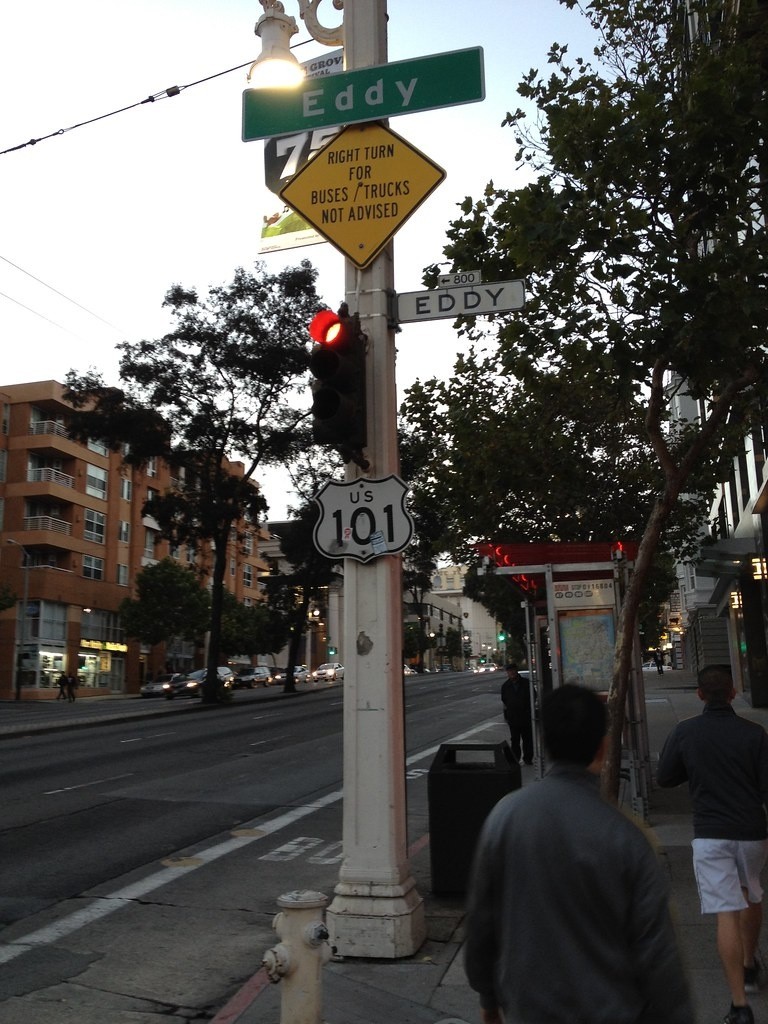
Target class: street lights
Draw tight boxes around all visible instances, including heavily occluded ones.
[306,605,320,671]
[248,1,429,961]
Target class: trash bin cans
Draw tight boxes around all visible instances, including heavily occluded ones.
[427,739,522,901]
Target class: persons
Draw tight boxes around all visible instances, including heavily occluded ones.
[66,673,79,703]
[542,663,553,693]
[654,649,664,676]
[56,672,67,700]
[146,667,153,684]
[501,664,537,765]
[465,683,698,1024]
[656,666,768,1024]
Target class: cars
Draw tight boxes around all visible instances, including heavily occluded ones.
[312,663,344,682]
[275,666,309,683]
[642,662,672,671]
[140,673,180,696]
[162,668,220,700]
[234,666,271,688]
[436,663,452,673]
[217,667,233,683]
[404,664,417,675]
[480,663,497,672]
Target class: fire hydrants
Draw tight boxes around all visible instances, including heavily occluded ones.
[261,890,338,1024]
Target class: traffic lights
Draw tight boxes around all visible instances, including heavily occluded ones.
[310,310,367,449]
[329,647,338,655]
[500,631,504,642]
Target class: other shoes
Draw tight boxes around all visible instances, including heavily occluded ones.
[744,957,761,990]
[525,759,533,765]
[722,1004,754,1024]
